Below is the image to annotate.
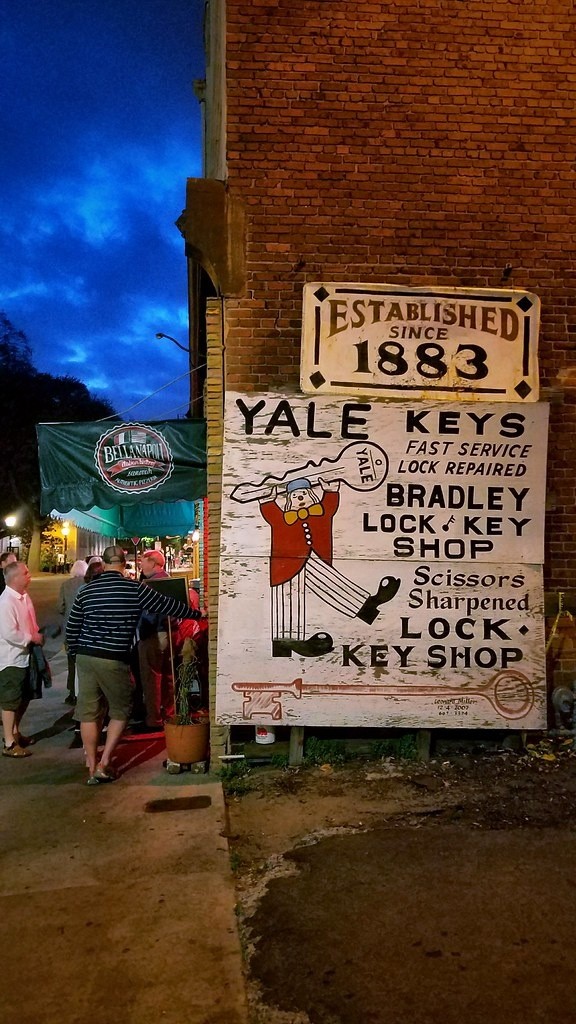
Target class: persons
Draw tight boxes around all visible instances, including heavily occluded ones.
[56,545,172,785]
[0,553,43,757]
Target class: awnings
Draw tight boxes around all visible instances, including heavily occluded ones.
[35,419,207,539]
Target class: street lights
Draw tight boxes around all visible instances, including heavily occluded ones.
[5,516,17,553]
[61,521,69,574]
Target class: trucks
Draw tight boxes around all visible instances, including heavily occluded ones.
[43,522,115,573]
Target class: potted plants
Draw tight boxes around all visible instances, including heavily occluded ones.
[163,640,210,763]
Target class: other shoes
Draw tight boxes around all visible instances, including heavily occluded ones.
[65,695,77,705]
[102,726,108,732]
[87,777,99,785]
[94,763,116,779]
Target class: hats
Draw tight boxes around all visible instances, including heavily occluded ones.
[104,546,124,564]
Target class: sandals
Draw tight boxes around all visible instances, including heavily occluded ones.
[12,733,34,745]
[2,744,32,757]
